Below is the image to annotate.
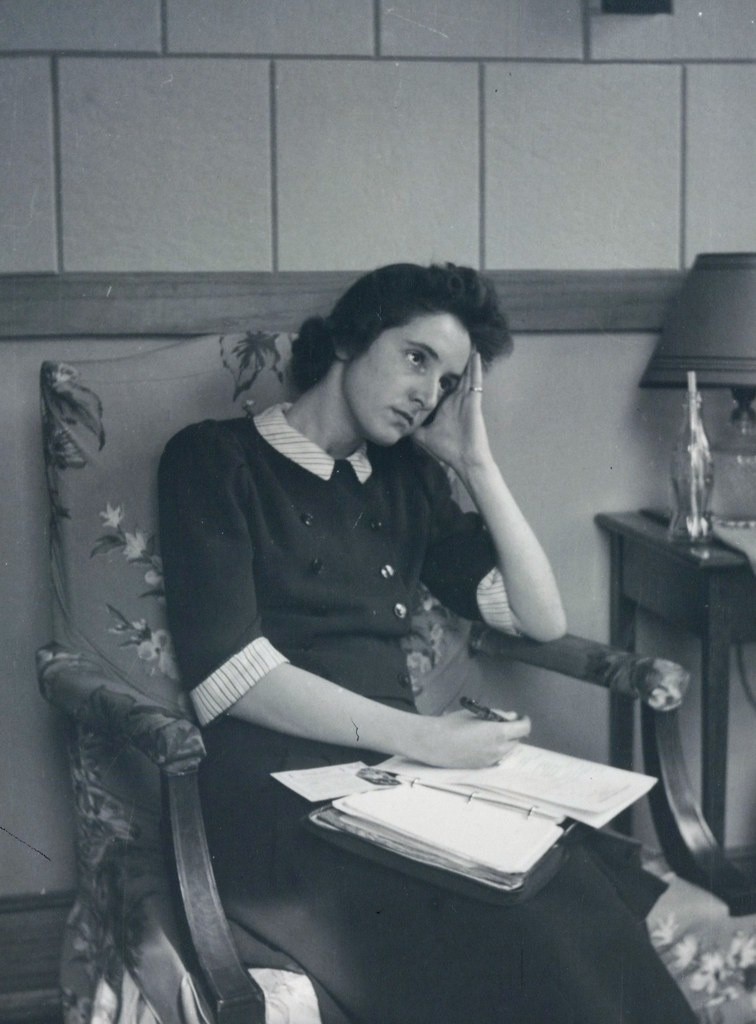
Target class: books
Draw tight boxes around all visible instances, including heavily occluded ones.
[310,742,660,908]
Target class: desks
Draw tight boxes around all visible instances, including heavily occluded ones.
[594,511,755,850]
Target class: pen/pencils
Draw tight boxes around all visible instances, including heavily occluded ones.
[460,697,510,723]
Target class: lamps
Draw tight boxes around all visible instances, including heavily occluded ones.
[640,253,756,528]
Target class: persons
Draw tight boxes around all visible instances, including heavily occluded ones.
[156,264,703,1024]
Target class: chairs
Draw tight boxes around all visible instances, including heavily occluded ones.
[31,321,755,1024]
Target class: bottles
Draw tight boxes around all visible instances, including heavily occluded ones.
[665,390,716,543]
